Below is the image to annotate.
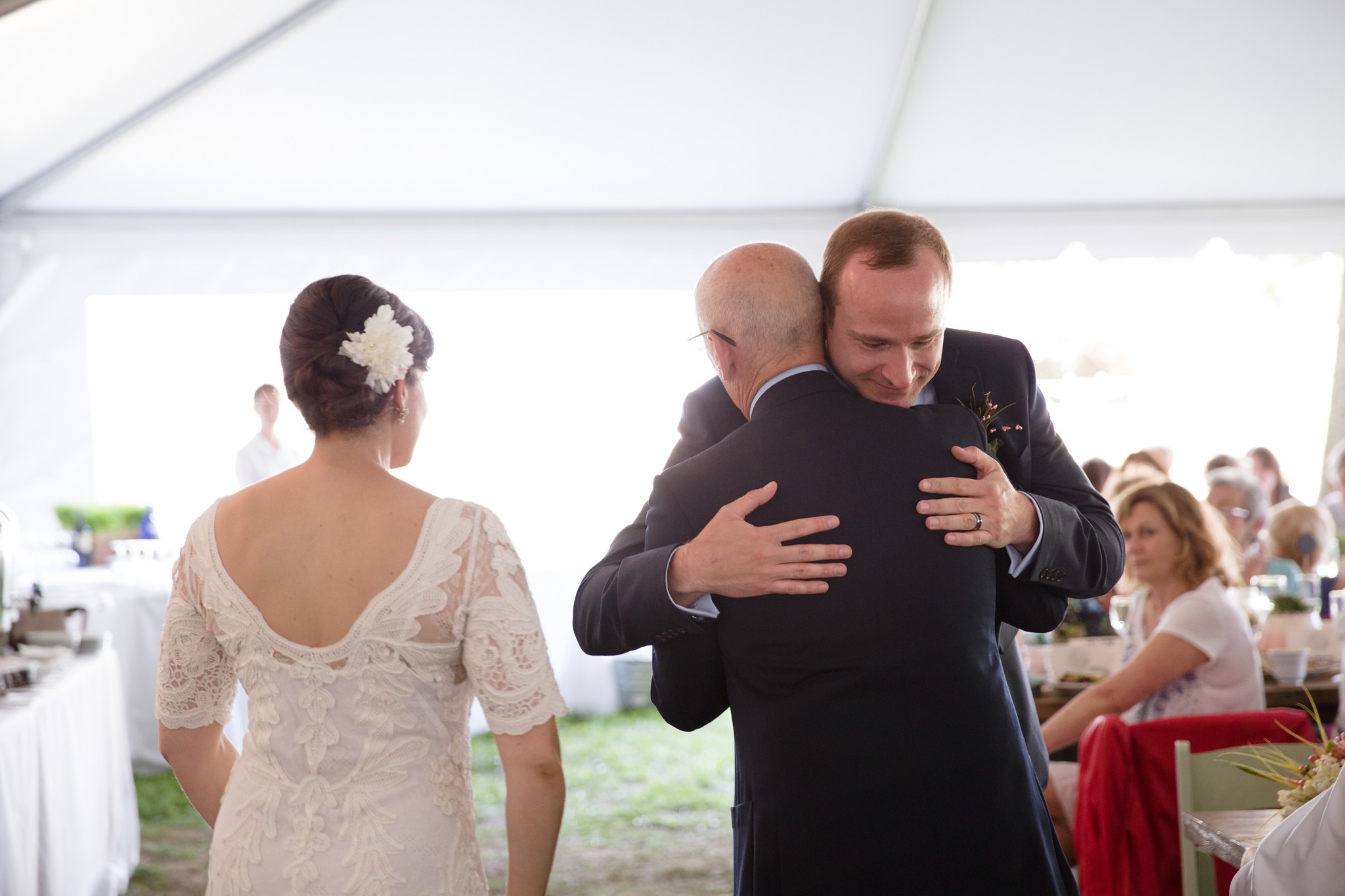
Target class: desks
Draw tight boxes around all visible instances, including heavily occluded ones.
[0,565,249,896]
[1184,809,1280,868]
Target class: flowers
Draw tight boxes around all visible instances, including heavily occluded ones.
[338,304,414,395]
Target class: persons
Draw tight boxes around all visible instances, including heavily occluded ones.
[574,207,1124,789]
[1019,444,1345,741]
[1229,759,1345,896]
[157,275,568,896]
[651,246,1078,896]
[1041,484,1266,858]
[235,384,284,490]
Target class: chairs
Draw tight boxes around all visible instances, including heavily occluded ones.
[1074,708,1325,896]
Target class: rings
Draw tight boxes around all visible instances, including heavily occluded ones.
[971,512,981,531]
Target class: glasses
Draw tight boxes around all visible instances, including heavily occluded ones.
[687,329,736,350]
